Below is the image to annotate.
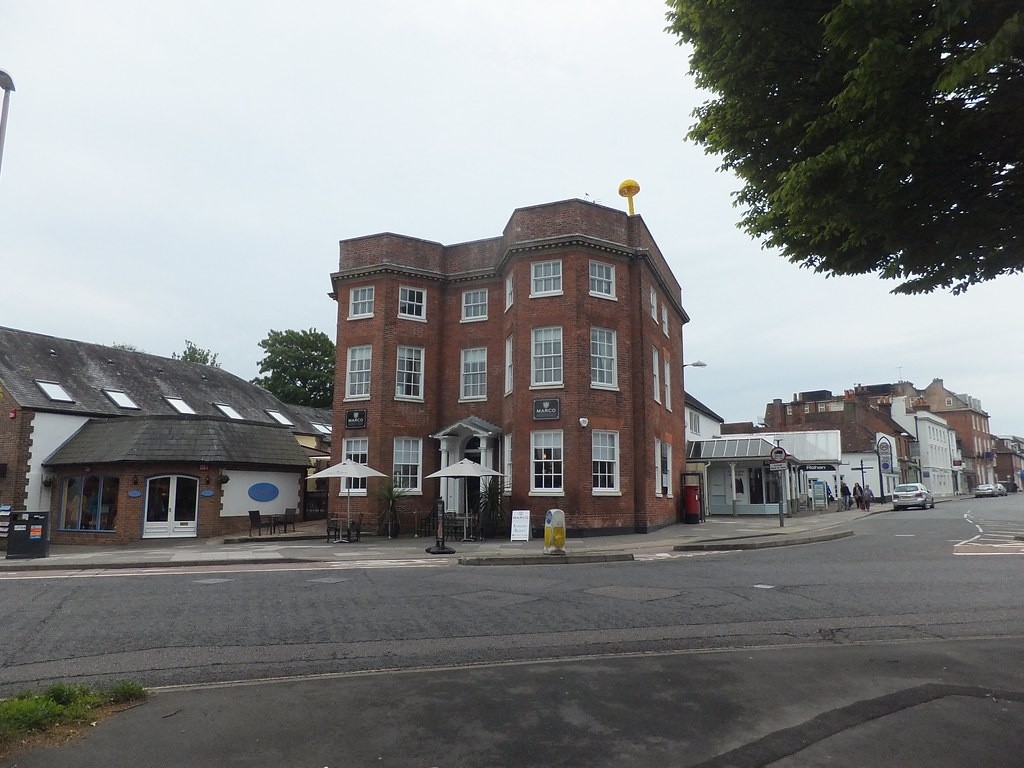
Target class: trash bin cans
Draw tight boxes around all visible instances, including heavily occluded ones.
[543,509,567,555]
[5,509,50,559]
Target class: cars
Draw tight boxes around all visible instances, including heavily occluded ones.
[974,484,998,497]
[892,483,934,510]
[993,484,1007,496]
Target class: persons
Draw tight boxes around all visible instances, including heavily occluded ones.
[862,485,874,511]
[853,482,863,508]
[841,483,851,511]
[826,482,831,503]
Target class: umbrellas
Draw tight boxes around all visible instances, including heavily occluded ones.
[423,458,507,527]
[305,459,389,527]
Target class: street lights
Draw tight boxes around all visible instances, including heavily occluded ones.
[775,438,783,526]
[901,433,922,483]
[0,70,15,168]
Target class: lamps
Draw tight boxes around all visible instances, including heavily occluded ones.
[206,475,209,484]
[134,475,138,485]
[42,473,57,487]
[683,361,707,368]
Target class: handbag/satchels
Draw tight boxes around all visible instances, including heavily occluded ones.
[828,495,834,501]
[861,501,865,510]
[854,494,858,499]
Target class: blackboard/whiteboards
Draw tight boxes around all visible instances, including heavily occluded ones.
[510,509,531,540]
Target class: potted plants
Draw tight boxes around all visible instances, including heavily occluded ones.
[466,472,515,539]
[371,474,412,537]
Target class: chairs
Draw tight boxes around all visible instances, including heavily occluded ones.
[327,513,340,544]
[248,510,272,536]
[348,512,364,542]
[274,507,296,534]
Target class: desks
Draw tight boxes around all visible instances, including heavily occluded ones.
[331,519,349,543]
